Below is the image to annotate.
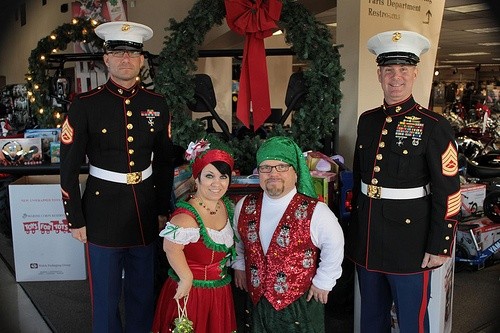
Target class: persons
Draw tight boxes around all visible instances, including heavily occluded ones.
[231,135,344,333]
[153,135,240,332]
[344,29,462,333]
[61,21,174,333]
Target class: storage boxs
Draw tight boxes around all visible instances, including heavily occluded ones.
[0,129,60,166]
[8,175,88,282]
[456,183,500,260]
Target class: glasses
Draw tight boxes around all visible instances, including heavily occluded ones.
[106,51,141,58]
[258,164,293,173]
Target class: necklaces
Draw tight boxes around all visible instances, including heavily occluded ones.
[189,192,222,215]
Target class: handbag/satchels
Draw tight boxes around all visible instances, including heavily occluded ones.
[172,294,194,333]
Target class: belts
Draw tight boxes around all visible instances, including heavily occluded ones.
[89,162,153,184]
[360,180,431,200]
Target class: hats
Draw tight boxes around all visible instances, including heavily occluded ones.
[94,21,153,52]
[184,131,234,180]
[365,30,431,67]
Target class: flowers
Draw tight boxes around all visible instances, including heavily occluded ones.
[185,138,211,164]
